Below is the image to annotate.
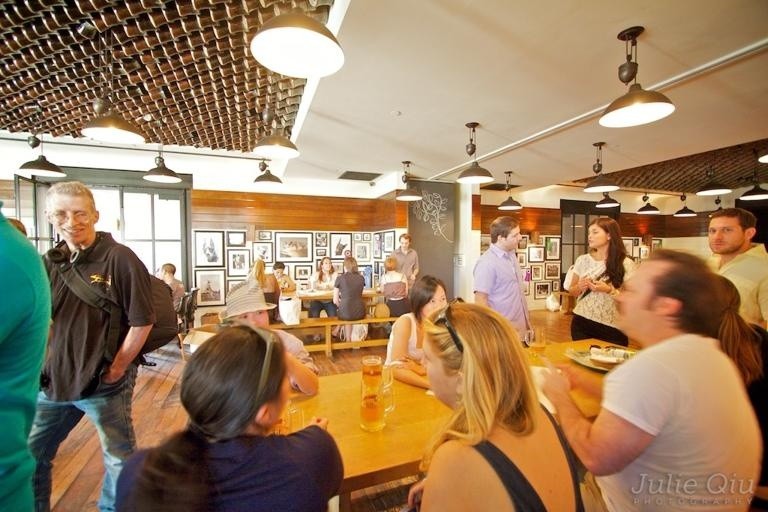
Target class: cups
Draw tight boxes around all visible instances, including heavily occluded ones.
[359,386,395,434]
[274,406,305,436]
[360,355,393,389]
[525,326,546,354]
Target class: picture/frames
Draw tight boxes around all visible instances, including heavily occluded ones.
[194,230,395,306]
[623,239,662,263]
[482,235,561,299]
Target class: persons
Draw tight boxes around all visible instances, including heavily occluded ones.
[540,248,764,512]
[28,181,157,512]
[472,215,535,348]
[160,263,185,311]
[569,217,638,347]
[710,275,768,512]
[706,208,768,331]
[0,199,52,512]
[6,218,27,236]
[115,324,345,512]
[408,304,585,512]
[222,234,447,397]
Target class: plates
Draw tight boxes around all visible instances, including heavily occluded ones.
[562,351,611,372]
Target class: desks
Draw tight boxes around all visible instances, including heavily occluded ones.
[272,337,650,512]
[281,288,380,313]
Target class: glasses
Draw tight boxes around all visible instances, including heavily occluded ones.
[244,324,279,402]
[434,297,464,352]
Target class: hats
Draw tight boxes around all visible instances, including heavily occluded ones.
[218,282,278,319]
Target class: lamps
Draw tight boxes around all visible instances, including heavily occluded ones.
[81,29,145,145]
[249,0,344,79]
[253,162,283,184]
[254,78,300,160]
[457,123,493,183]
[142,123,182,184]
[696,154,768,200]
[17,109,67,178]
[584,142,620,193]
[396,162,422,202]
[598,26,676,129]
[596,191,697,218]
[497,171,522,211]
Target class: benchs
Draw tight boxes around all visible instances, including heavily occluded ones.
[272,316,400,357]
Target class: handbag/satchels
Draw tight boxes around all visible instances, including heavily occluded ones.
[351,324,368,349]
[140,275,178,354]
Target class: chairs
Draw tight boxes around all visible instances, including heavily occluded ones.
[183,289,200,334]
[175,294,191,334]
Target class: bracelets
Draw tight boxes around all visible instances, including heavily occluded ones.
[607,286,615,297]
